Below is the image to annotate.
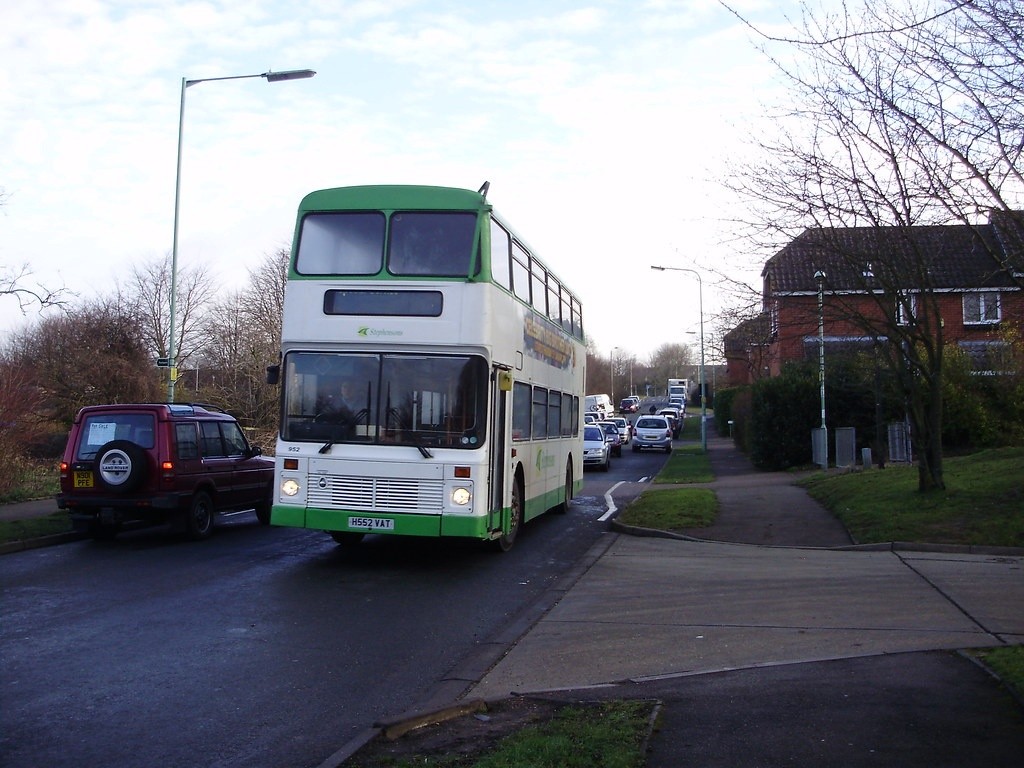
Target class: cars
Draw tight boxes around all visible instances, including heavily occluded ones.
[589,421,621,456]
[584,411,607,425]
[619,398,636,412]
[659,398,685,435]
[628,395,641,410]
[603,418,631,445]
[582,424,610,472]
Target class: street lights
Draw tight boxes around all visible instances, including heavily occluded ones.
[814,270,826,428]
[164,68,317,401]
[650,264,708,453]
[686,331,715,413]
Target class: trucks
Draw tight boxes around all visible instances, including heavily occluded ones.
[668,379,689,405]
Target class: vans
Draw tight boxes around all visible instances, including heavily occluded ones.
[586,394,614,418]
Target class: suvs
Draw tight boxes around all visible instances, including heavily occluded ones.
[632,415,673,454]
[54,405,274,535]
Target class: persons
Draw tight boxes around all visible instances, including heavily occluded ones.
[649,404,657,413]
[629,421,634,435]
[322,380,362,423]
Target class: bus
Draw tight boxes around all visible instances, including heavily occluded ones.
[271,182,587,558]
